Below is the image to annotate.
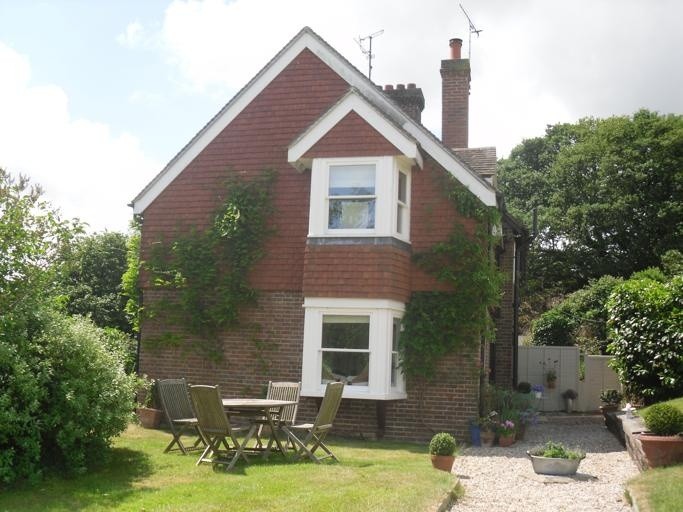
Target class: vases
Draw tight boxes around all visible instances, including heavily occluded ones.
[535,391,542,399]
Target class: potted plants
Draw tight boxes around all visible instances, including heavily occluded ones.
[637,403,683,468]
[429,432,456,473]
[472,381,532,447]
[526,441,586,475]
[599,389,624,426]
[137,378,164,429]
[562,389,578,414]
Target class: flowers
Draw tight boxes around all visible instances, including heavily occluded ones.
[531,384,545,392]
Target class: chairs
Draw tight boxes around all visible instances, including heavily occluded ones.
[157,377,344,472]
